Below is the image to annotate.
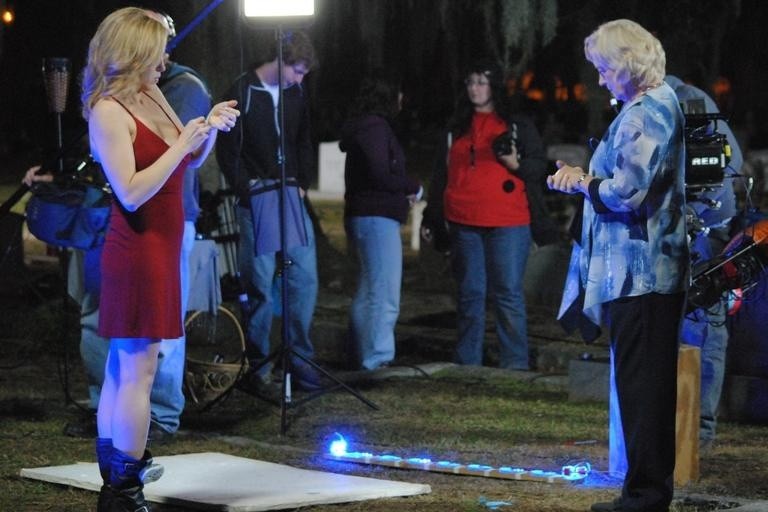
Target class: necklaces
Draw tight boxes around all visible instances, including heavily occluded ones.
[468,111,489,167]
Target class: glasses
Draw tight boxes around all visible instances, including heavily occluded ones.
[463,78,489,88]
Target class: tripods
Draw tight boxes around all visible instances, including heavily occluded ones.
[204,26,380,431]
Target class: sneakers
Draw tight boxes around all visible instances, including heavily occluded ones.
[291,362,321,391]
[245,374,281,399]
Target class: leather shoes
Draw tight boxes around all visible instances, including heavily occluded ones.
[589,495,672,511]
[63,408,97,437]
[147,421,174,446]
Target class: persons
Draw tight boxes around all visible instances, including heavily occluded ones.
[216,32,327,398]
[339,78,423,372]
[546,19,689,511]
[79,6,243,512]
[421,62,560,371]
[662,74,743,462]
[22,12,213,441]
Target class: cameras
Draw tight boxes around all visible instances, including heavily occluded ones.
[493,131,525,159]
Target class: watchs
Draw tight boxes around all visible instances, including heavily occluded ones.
[577,174,588,191]
